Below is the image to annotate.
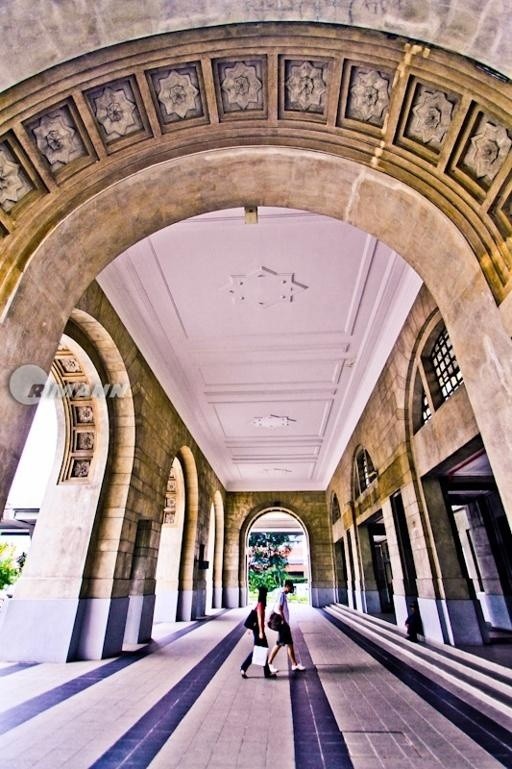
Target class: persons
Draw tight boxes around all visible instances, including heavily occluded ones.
[240,587,277,680]
[268,579,306,673]
[405,600,424,642]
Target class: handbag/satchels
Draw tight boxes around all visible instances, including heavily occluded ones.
[244,610,258,632]
[268,612,282,631]
[251,645,269,667]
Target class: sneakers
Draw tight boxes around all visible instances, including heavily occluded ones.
[265,665,278,679]
[240,670,247,679]
[292,664,306,670]
